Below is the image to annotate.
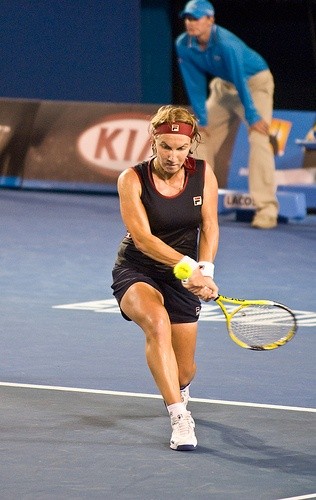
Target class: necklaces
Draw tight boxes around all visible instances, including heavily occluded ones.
[163,167,184,190]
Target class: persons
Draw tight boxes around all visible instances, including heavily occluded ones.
[176,0,279,229]
[111,104,218,451]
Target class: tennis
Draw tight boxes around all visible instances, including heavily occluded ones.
[174,262,191,280]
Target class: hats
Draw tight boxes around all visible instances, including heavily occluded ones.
[180,0,214,20]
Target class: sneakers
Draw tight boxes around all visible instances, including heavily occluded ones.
[251,213,278,228]
[169,411,197,450]
[164,384,190,415]
